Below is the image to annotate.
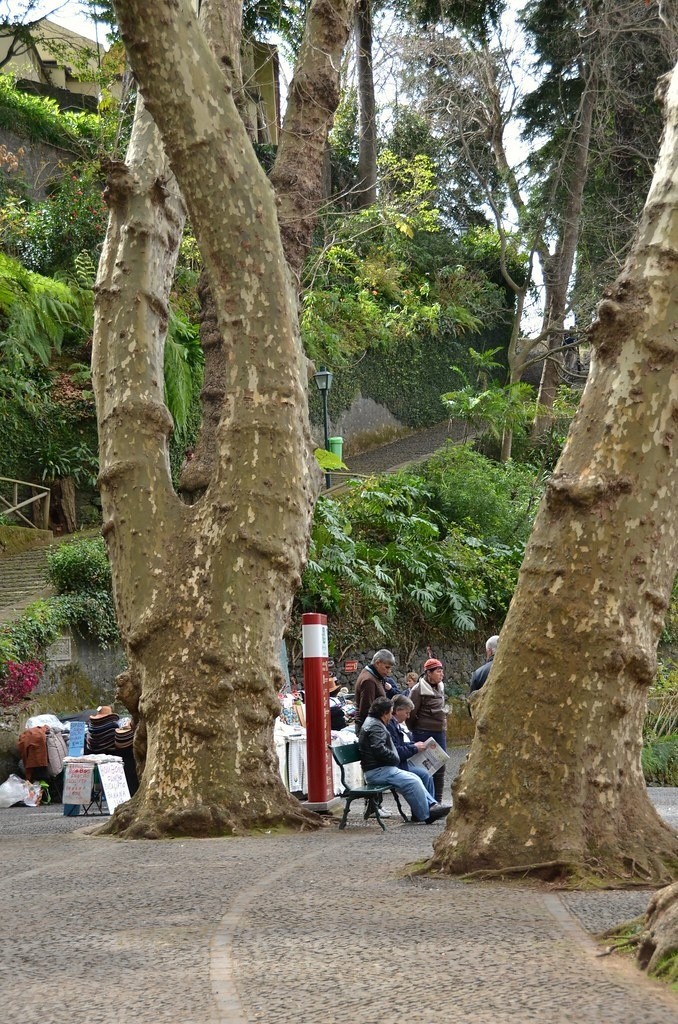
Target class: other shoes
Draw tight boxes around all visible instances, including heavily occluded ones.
[362,808,393,818]
[425,805,452,824]
[381,807,393,813]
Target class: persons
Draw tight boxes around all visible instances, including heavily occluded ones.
[405,658,446,823]
[358,694,452,826]
[354,648,396,818]
[470,635,500,693]
[384,671,419,699]
[426,644,434,658]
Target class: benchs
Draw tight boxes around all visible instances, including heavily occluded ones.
[326,741,409,831]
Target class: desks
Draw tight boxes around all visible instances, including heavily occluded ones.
[63,763,124,816]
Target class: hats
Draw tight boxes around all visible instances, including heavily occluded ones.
[424,658,442,670]
[329,678,341,693]
[86,706,135,751]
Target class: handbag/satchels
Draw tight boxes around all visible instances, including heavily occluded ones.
[46,730,68,777]
